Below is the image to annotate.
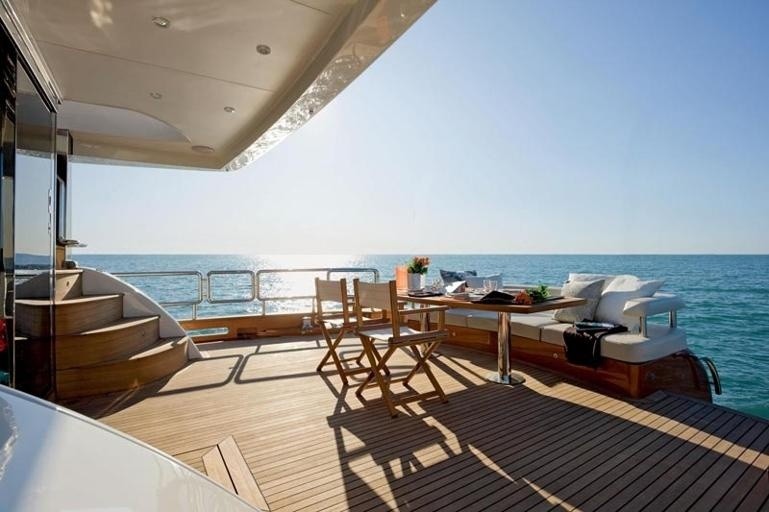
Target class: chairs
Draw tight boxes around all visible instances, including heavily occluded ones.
[353,278,449,418]
[315,277,390,385]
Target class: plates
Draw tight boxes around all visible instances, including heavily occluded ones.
[448,292,469,297]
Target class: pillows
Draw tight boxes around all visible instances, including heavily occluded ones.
[440,269,477,287]
[466,274,502,289]
[569,273,616,291]
[552,280,604,323]
[595,275,665,331]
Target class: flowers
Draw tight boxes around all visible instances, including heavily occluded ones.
[407,256,430,275]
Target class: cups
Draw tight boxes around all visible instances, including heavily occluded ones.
[432,278,441,294]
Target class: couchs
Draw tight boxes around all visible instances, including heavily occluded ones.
[403,284,687,399]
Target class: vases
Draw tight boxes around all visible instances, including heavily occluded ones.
[407,273,425,289]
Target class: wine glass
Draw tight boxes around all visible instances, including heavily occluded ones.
[483,279,497,293]
